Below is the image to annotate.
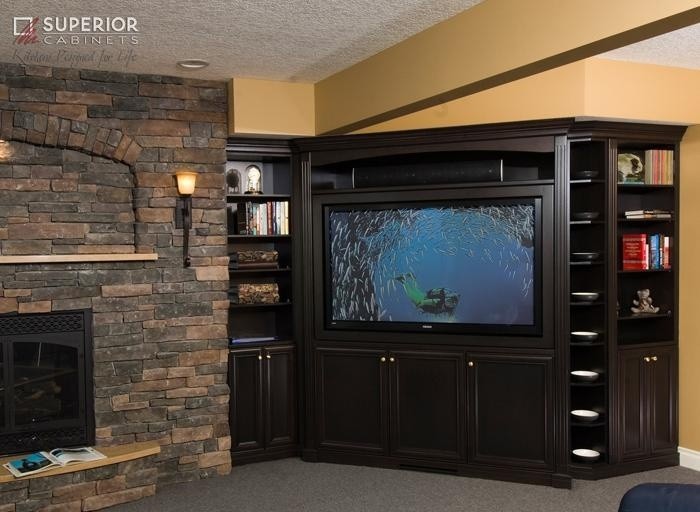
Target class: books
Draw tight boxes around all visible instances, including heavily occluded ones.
[228,250,279,305]
[621,149,674,271]
[2,446,108,479]
[235,199,289,236]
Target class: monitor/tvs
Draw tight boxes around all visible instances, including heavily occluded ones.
[322,197,543,337]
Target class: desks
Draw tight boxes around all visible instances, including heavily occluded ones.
[0,440,163,512]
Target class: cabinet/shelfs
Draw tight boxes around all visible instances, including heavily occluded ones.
[315,341,554,484]
[226,141,302,466]
[562,132,680,482]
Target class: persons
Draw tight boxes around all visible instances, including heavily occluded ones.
[395,271,460,316]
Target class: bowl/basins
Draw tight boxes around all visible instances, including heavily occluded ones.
[573,211,600,222]
[570,409,600,424]
[571,371,599,383]
[571,291,600,303]
[571,448,601,464]
[570,170,599,179]
[571,252,599,261]
[570,331,598,344]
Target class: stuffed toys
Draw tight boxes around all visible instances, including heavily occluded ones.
[630,289,660,315]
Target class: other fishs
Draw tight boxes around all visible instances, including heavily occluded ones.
[330,205,534,322]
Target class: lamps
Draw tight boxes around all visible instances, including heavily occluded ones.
[174,172,196,269]
